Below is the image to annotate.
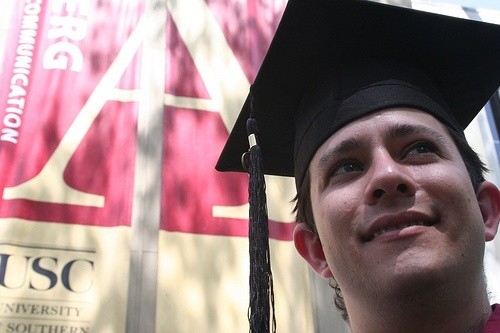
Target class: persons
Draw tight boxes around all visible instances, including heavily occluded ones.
[214,0,500,333]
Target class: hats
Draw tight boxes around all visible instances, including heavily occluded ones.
[213,0,500,333]
